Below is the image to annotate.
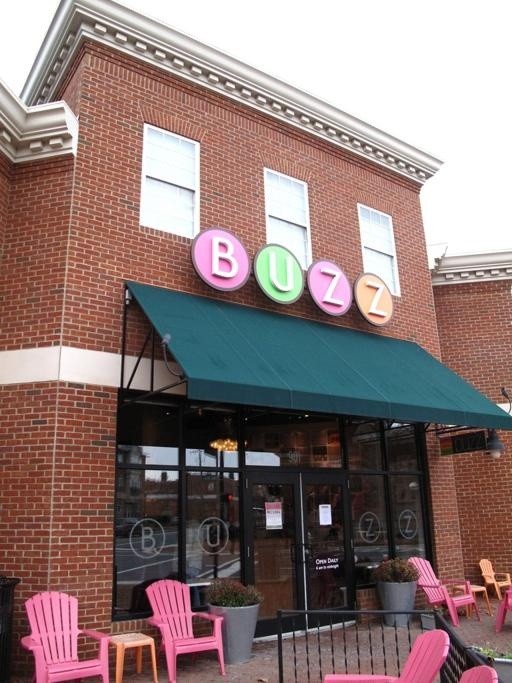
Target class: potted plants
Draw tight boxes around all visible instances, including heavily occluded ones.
[208,577,262,664]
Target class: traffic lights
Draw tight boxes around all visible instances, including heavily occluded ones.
[223,493,233,502]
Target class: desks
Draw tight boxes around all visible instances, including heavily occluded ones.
[107,632,158,682]
[455,585,493,619]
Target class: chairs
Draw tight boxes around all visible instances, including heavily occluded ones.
[20,591,112,683]
[477,559,511,600]
[409,556,481,627]
[145,579,225,682]
[494,584,512,633]
[458,665,499,683]
[322,628,450,683]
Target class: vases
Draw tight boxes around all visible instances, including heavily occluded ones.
[375,558,420,628]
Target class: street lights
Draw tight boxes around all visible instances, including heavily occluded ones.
[202,443,219,578]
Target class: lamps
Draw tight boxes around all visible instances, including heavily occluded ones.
[485,430,507,460]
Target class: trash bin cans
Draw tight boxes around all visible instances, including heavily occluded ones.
[0,576,20,683]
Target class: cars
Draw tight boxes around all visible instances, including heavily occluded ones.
[117,516,143,537]
[170,515,179,524]
